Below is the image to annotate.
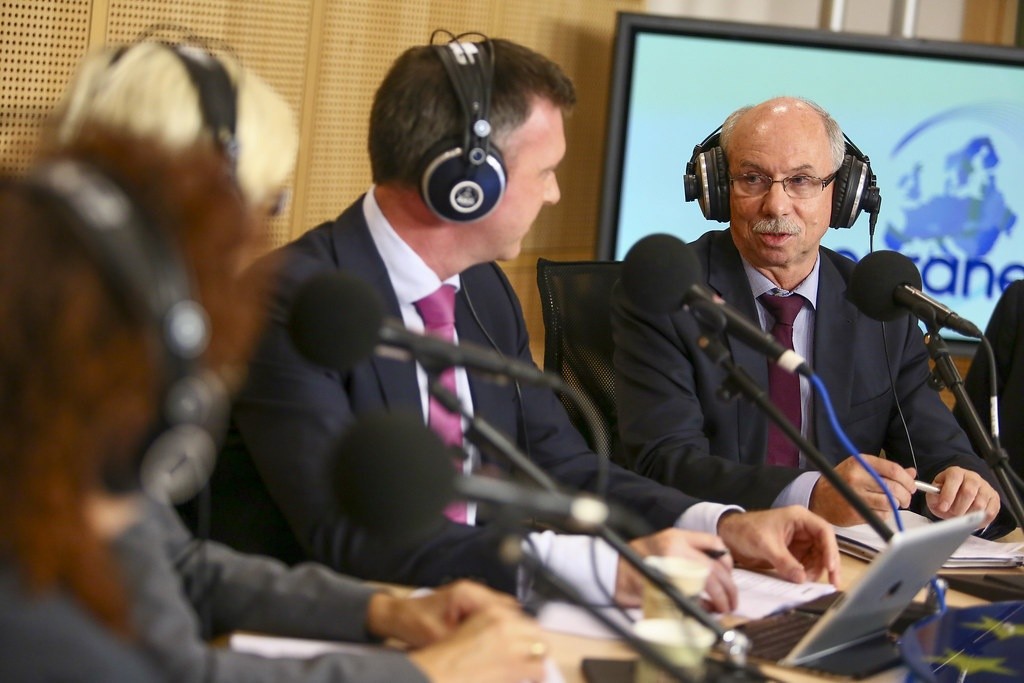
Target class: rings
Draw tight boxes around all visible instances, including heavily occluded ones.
[531,636,548,666]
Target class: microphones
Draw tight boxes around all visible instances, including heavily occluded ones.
[848,250,986,339]
[326,405,612,549]
[286,270,567,391]
[621,233,813,379]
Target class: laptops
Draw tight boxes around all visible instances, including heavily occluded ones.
[732,512,986,669]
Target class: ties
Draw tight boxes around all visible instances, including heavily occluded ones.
[413,283,467,524]
[758,291,805,471]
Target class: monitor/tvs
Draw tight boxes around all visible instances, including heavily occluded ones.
[593,8,1024,358]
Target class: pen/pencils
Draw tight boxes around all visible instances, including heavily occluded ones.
[913,480,943,495]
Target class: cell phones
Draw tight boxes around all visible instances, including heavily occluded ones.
[985,574,1024,591]
[796,591,936,637]
[581,658,638,683]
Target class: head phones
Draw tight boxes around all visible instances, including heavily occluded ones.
[0,153,226,514]
[418,26,509,228]
[684,123,882,232]
[104,24,252,250]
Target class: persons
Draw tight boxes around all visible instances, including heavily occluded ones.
[948,278,1024,539]
[1,140,556,683]
[608,95,1019,548]
[227,31,844,614]
[24,28,246,680]
